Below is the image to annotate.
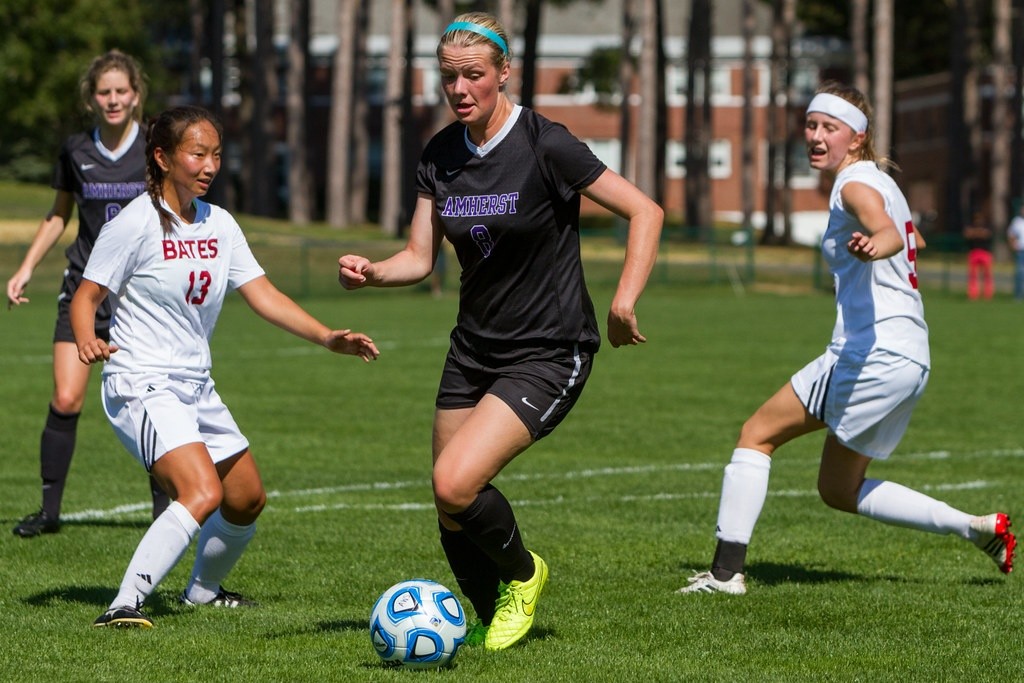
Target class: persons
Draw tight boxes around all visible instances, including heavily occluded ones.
[64,103,381,632]
[336,8,667,659]
[676,82,1019,598]
[959,205,1024,299]
[4,50,175,538]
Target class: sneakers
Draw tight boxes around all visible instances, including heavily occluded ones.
[181,587,260,611]
[669,570,746,599]
[485,549,549,651]
[458,617,491,648]
[93,606,153,629]
[15,511,63,536]
[971,512,1017,576]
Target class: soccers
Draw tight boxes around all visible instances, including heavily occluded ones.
[367,577,470,672]
